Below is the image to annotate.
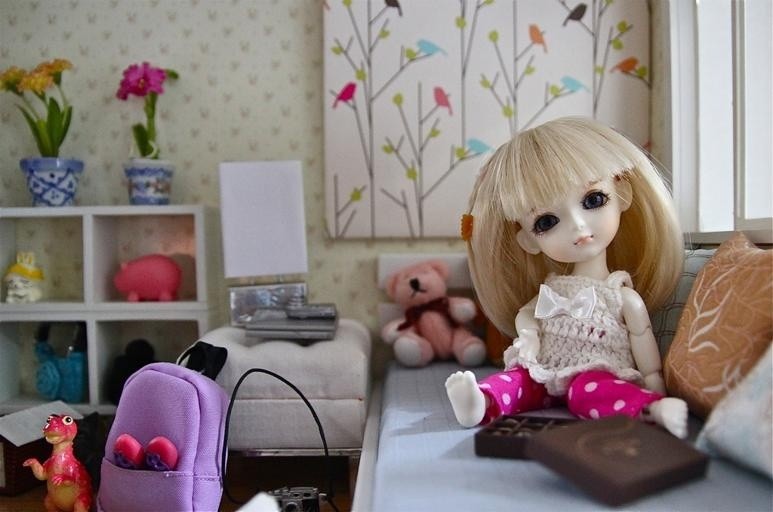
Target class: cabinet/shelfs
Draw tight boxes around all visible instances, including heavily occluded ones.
[0,201,211,418]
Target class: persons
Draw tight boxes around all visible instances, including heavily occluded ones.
[442,116,689,440]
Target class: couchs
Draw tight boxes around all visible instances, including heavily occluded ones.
[346,239,773,512]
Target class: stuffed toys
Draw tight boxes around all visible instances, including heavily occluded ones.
[379,258,487,365]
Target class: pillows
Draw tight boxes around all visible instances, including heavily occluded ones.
[654,229,773,482]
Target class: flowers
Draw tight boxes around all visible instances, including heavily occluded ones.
[0,56,75,158]
[115,59,181,162]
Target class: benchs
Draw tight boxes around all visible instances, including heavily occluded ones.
[178,309,371,502]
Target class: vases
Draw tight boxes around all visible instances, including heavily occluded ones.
[124,165,176,205]
[20,159,83,208]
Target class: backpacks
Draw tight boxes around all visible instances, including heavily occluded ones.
[95,340,231,512]
